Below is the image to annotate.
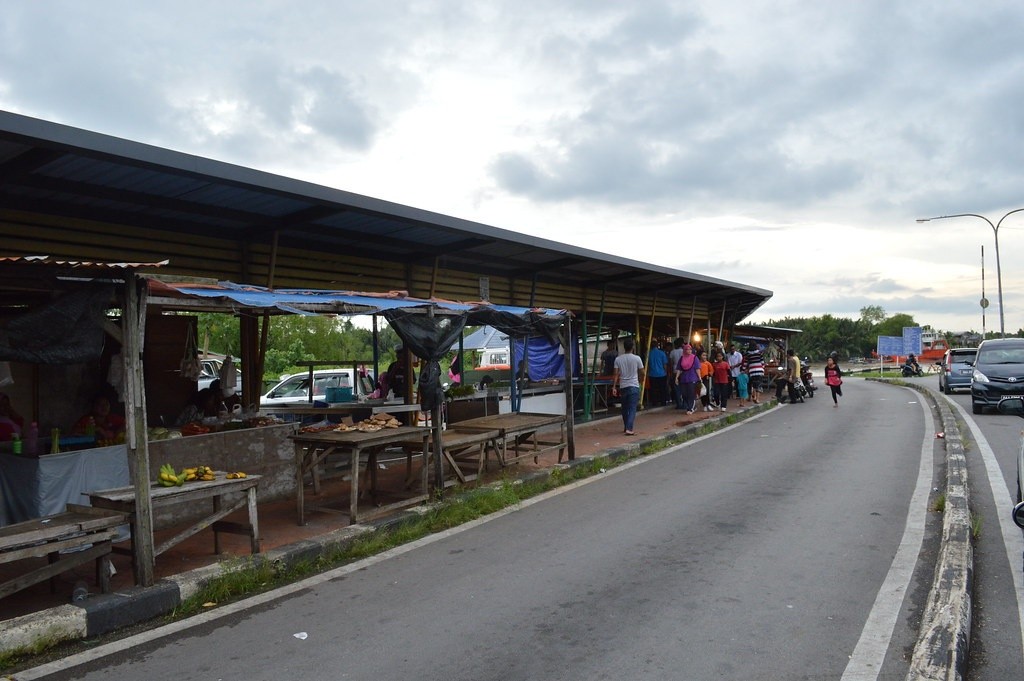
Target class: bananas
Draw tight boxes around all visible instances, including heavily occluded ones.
[157,463,247,487]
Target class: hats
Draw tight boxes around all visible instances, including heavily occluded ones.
[393,344,403,351]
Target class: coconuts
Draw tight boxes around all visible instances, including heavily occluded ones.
[147,427,182,441]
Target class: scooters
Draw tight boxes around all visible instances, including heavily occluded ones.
[900,360,924,377]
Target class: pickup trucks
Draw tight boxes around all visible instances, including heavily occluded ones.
[260,368,446,430]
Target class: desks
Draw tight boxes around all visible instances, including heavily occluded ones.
[572,380,614,420]
[259,405,420,473]
[446,412,567,471]
[285,423,437,525]
[391,429,499,489]
[80,470,263,567]
[0,503,132,600]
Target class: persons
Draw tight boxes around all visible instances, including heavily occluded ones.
[0,392,24,441]
[674,345,701,415]
[698,351,714,411]
[600,340,618,375]
[824,357,843,407]
[663,337,705,410]
[772,349,801,399]
[906,354,916,372]
[777,346,785,367]
[648,341,668,406]
[710,340,766,407]
[712,353,734,412]
[175,379,241,424]
[380,348,417,398]
[71,396,125,438]
[611,340,646,435]
[516,360,529,379]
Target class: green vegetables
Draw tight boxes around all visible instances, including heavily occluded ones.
[444,384,474,400]
[488,381,512,387]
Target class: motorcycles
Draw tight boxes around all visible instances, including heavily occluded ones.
[777,357,818,403]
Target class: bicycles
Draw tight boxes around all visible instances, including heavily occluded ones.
[927,361,941,374]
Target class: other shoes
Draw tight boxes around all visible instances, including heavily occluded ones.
[755,399,759,404]
[708,405,714,411]
[739,403,745,407]
[704,406,707,412]
[753,399,756,403]
[694,406,697,412]
[716,405,720,411]
[687,410,693,415]
[834,403,838,408]
[789,399,797,404]
[626,430,637,435]
[722,407,729,412]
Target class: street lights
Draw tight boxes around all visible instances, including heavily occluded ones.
[916,209,1024,337]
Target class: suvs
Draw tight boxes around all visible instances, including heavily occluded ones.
[965,338,1024,414]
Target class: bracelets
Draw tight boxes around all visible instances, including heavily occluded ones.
[612,388,616,389]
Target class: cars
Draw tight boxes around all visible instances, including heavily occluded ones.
[935,347,978,394]
[197,359,242,397]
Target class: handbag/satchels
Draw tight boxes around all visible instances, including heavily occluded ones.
[180,359,204,381]
[696,380,707,398]
